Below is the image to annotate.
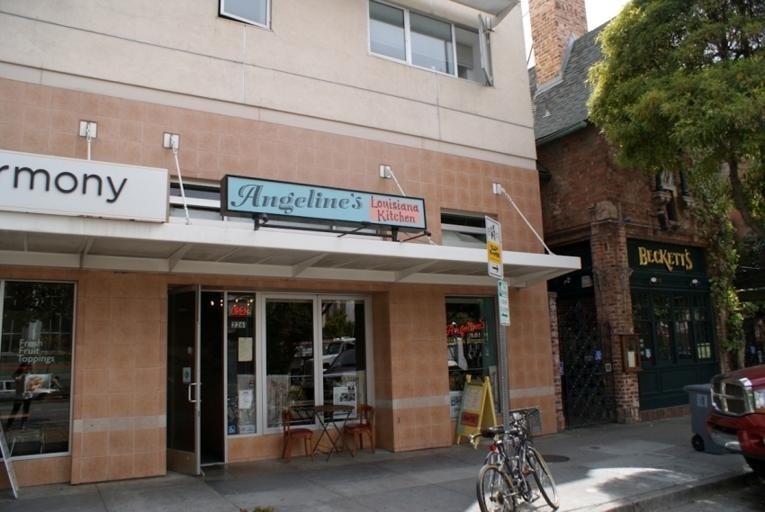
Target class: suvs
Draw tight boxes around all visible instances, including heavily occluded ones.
[705,363,765,482]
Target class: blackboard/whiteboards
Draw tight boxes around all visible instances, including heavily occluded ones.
[456,385,497,438]
[0,424,19,499]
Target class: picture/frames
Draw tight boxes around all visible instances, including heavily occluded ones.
[618,333,646,373]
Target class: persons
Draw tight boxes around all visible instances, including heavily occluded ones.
[5,362,34,430]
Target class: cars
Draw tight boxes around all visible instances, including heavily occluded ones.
[295,336,356,401]
[0,380,61,403]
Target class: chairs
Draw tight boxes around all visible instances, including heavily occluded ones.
[281,402,376,462]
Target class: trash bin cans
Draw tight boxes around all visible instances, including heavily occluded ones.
[683,384,727,455]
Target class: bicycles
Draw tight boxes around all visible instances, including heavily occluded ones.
[468,408,559,512]
